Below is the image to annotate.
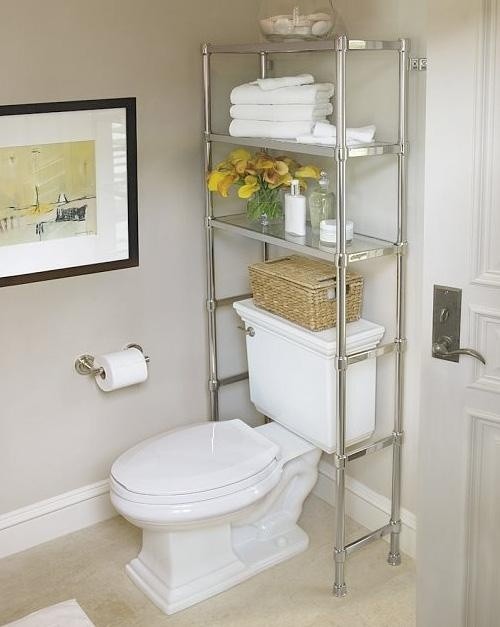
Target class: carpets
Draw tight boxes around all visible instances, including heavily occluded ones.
[4,600,96,627]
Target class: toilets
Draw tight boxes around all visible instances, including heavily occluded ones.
[108,298,386,617]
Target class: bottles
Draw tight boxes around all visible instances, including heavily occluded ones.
[260,210,267,226]
[257,0,337,45]
[308,169,336,237]
[283,180,307,237]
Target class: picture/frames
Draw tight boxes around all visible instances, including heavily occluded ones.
[0,97,140,288]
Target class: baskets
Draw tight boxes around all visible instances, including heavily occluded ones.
[246,254,365,334]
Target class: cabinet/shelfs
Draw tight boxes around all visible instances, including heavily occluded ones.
[204,35,411,599]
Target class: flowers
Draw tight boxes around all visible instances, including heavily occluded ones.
[206,147,322,219]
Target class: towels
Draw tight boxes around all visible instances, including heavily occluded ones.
[226,75,377,145]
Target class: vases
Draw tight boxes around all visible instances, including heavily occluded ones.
[246,190,283,223]
